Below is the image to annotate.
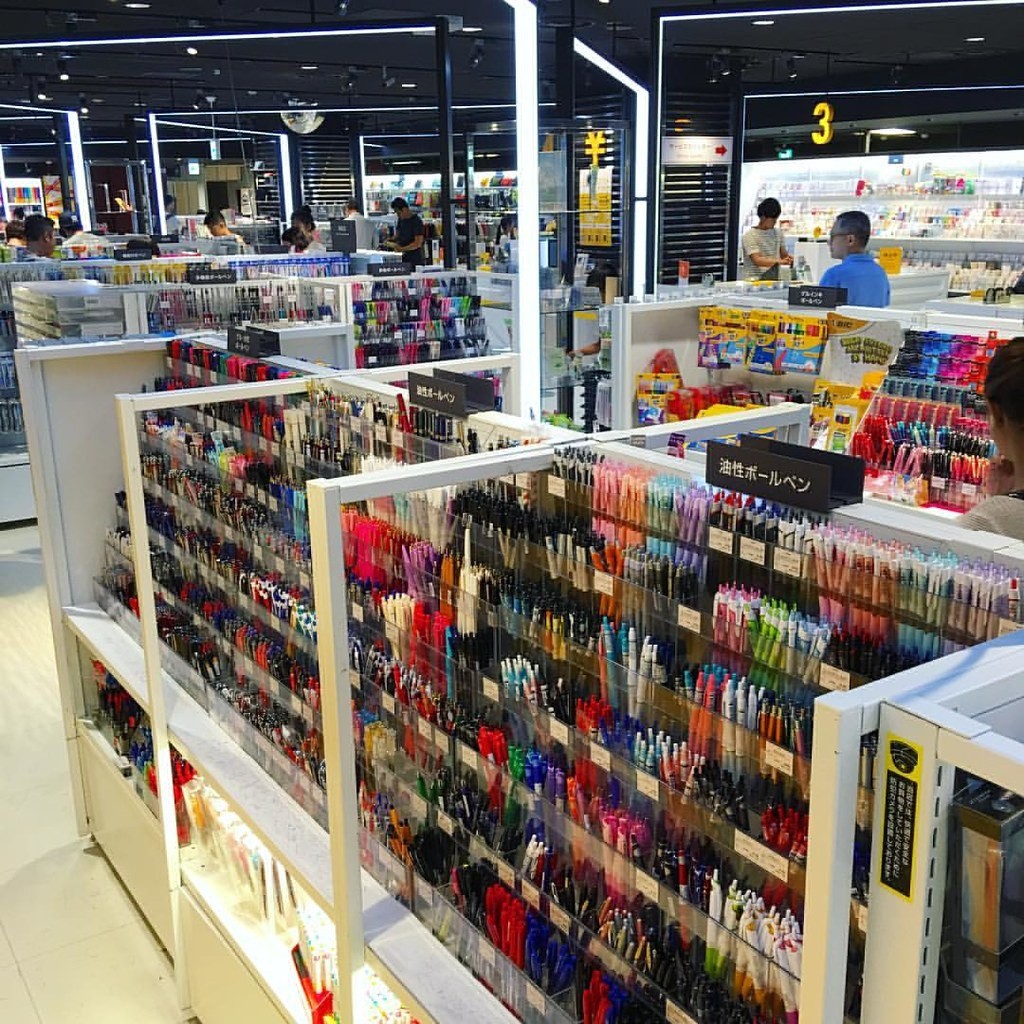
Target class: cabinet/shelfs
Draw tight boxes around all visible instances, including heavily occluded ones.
[2,180,1024,1023]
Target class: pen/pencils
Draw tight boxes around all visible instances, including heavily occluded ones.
[0,249,1021,1023]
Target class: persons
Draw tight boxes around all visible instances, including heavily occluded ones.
[14,208,24,221]
[291,204,326,253]
[154,195,182,240]
[5,221,27,246]
[17,214,57,262]
[742,198,793,282]
[564,261,621,356]
[495,219,516,247]
[204,210,244,244]
[384,197,425,266]
[817,211,890,309]
[59,211,110,244]
[951,334,1024,541]
[341,197,380,250]
[282,229,307,248]
